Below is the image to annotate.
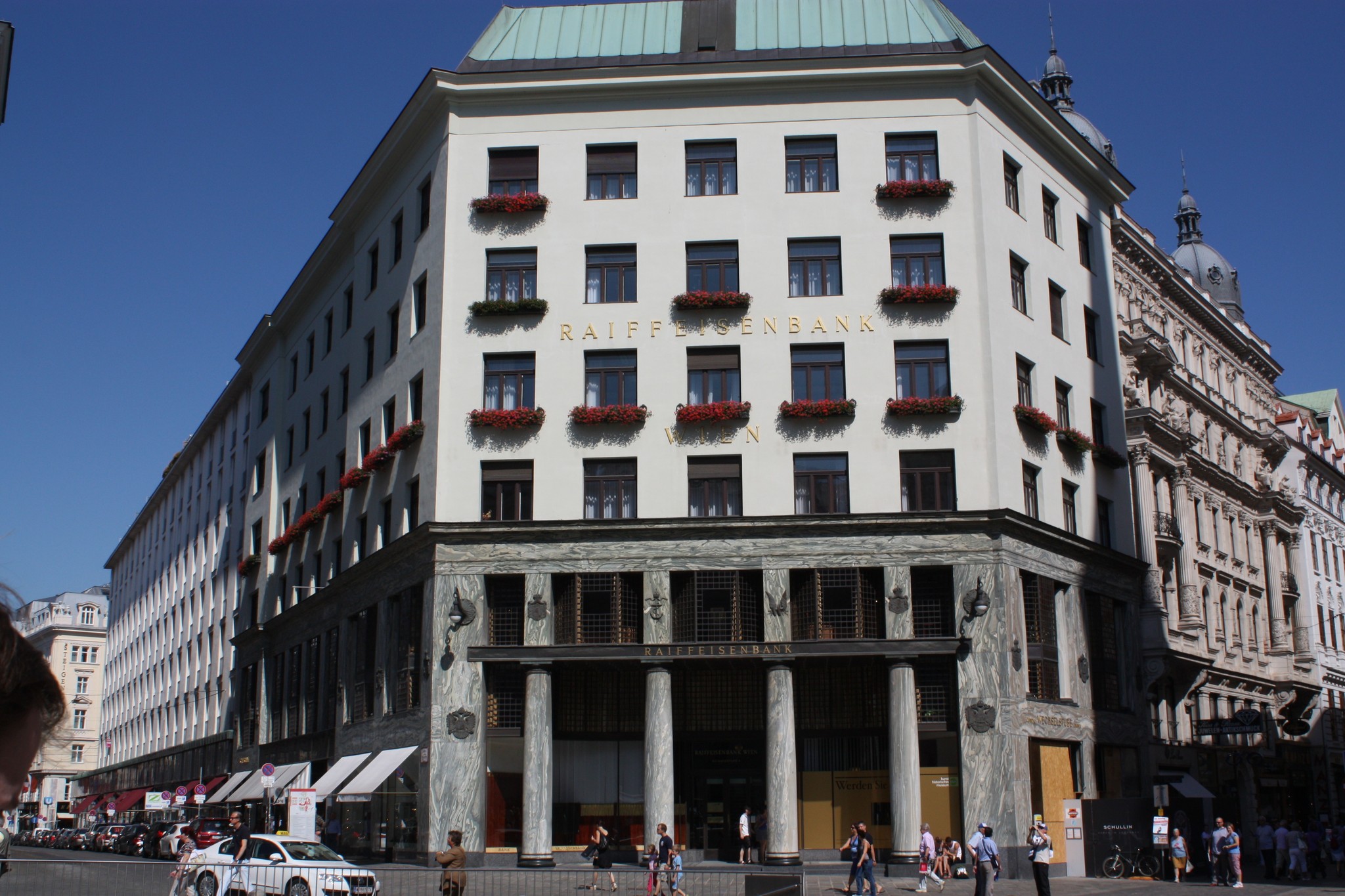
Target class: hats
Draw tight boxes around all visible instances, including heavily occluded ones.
[994,869,999,881]
[978,823,987,828]
[1036,824,1047,830]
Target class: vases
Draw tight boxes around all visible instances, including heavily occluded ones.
[1017,413,1049,434]
[677,304,749,309]
[372,455,395,469]
[879,192,951,196]
[477,205,547,212]
[888,297,956,302]
[473,310,546,315]
[1055,432,1084,451]
[396,434,421,448]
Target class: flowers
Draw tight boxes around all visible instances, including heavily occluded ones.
[675,290,752,422]
[339,419,426,489]
[1013,404,1097,452]
[569,404,653,427]
[267,490,344,555]
[237,554,260,580]
[779,400,856,418]
[876,180,964,414]
[465,190,553,430]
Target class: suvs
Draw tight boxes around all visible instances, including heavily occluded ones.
[156,823,190,861]
[138,821,187,859]
[10,822,126,853]
[114,823,150,856]
[177,816,236,852]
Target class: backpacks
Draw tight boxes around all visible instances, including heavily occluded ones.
[953,865,969,879]
[1330,835,1340,849]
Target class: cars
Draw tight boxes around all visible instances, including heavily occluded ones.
[187,831,381,896]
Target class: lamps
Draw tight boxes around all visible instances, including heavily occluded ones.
[963,576,990,617]
[73,695,80,703]
[448,586,477,626]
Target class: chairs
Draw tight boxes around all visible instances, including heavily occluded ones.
[257,841,272,859]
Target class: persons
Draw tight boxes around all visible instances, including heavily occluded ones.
[737,804,768,865]
[915,823,945,893]
[590,819,617,892]
[215,811,254,896]
[433,830,467,896]
[325,811,341,854]
[966,822,1002,896]
[1026,823,1053,896]
[167,826,207,896]
[1200,817,1244,888]
[646,823,690,896]
[1249,813,1345,881]
[0,580,66,820]
[931,836,962,879]
[839,821,882,896]
[1167,828,1191,884]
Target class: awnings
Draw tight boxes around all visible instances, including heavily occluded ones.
[68,744,419,814]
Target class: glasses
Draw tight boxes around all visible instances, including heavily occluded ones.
[229,817,239,820]
[851,827,855,829]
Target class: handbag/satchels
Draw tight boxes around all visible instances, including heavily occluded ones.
[921,853,930,860]
[1298,838,1308,850]
[187,849,205,873]
[1028,847,1036,861]
[991,858,999,868]
[596,830,609,853]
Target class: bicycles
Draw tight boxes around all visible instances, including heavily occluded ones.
[1102,844,1161,879]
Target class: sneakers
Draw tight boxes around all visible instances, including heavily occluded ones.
[1209,883,1243,888]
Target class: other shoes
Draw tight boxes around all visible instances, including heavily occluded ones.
[590,884,596,890]
[739,861,746,864]
[915,889,927,893]
[611,883,617,891]
[942,874,952,879]
[747,860,752,864]
[940,881,945,891]
[1174,877,1179,883]
[842,886,886,896]
[1263,872,1345,880]
